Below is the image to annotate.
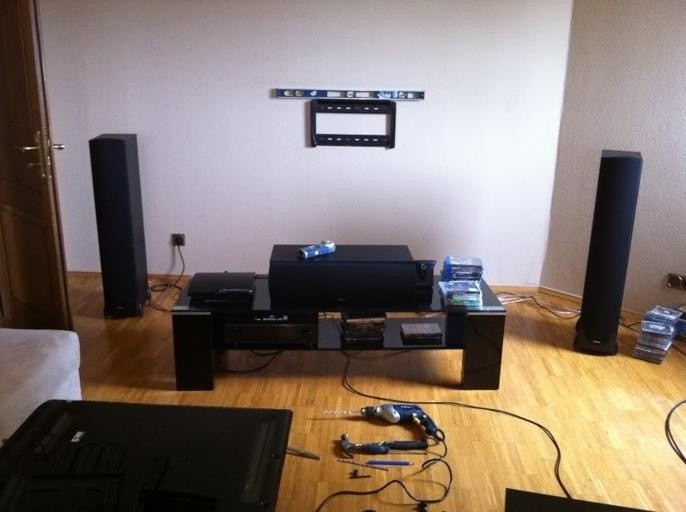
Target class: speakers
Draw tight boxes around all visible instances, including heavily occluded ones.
[268,245,417,312]
[88,133,148,317]
[573,149,643,357]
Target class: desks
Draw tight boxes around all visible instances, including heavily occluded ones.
[1,399,293,511]
[173,275,507,392]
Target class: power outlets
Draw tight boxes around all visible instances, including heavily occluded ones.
[171,234,185,246]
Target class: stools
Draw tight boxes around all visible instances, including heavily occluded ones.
[0,327,83,442]
